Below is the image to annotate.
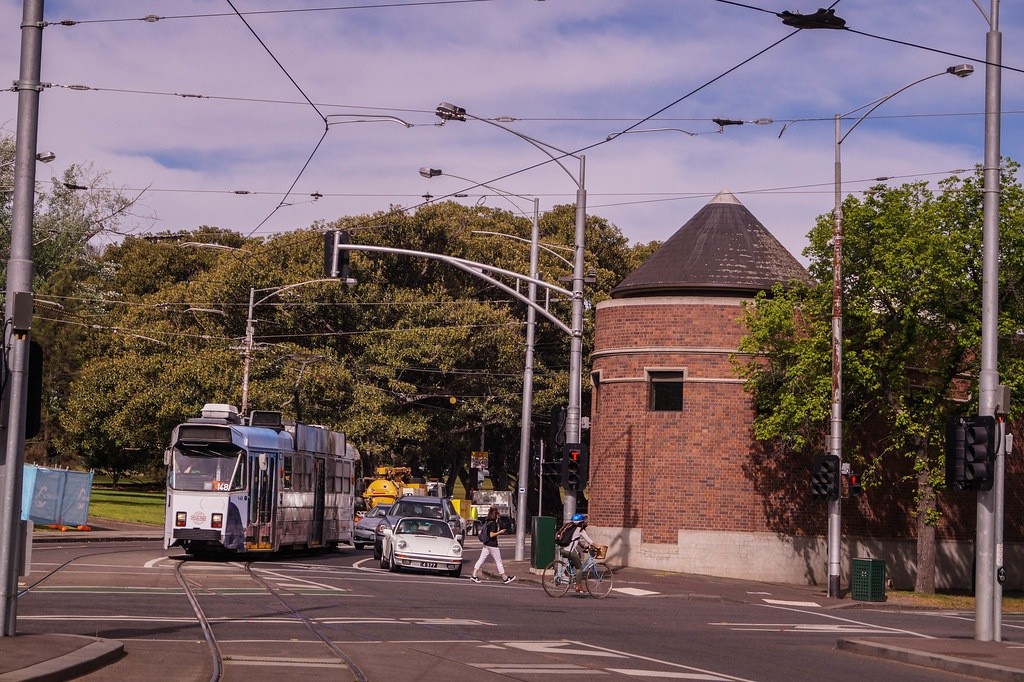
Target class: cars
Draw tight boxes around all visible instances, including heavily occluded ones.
[354,505,394,549]
[381,517,465,578]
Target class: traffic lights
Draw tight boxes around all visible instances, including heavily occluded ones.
[849,473,861,498]
[560,442,586,492]
[443,395,461,405]
[964,416,995,492]
[542,451,563,486]
[533,455,539,473]
[812,455,840,501]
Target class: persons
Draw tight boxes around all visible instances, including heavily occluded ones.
[182,460,202,473]
[470,506,516,585]
[555,514,601,592]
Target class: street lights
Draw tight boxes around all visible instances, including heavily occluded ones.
[240,278,359,424]
[419,167,539,561]
[436,104,585,580]
[829,60,974,599]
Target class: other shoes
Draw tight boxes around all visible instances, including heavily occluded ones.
[575,582,586,593]
[564,566,571,577]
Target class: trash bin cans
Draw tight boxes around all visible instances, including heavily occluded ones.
[851,558,888,602]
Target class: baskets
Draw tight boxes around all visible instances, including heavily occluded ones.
[592,544,608,559]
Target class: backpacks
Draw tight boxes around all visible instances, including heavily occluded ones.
[554,522,583,547]
[477,521,494,543]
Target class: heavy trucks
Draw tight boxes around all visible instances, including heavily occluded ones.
[463,490,518,535]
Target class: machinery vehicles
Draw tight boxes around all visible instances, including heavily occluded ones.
[354,465,452,527]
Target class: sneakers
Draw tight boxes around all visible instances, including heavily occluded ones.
[503,575,516,584]
[470,575,481,584]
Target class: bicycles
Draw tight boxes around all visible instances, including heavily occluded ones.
[541,544,615,599]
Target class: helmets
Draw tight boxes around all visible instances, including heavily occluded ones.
[571,514,584,526]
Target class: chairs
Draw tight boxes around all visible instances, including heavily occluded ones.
[401,523,417,533]
[428,525,441,537]
[401,504,442,518]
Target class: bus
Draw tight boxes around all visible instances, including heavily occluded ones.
[163,403,355,558]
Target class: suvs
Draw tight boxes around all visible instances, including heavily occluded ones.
[375,495,464,561]
